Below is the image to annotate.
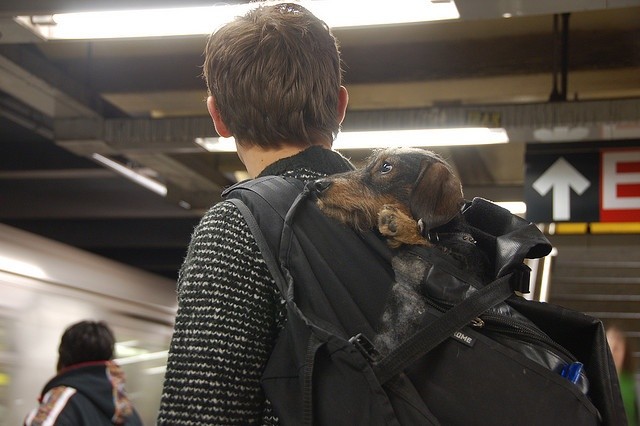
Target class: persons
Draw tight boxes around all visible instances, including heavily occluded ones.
[155,1,356,425]
[606,326,640,425]
[24,320,143,425]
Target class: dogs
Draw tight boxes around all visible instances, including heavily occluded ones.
[314,146,463,248]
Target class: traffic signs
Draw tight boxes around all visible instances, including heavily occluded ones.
[520,143,640,236]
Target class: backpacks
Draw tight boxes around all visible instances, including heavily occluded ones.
[222,176,629,426]
